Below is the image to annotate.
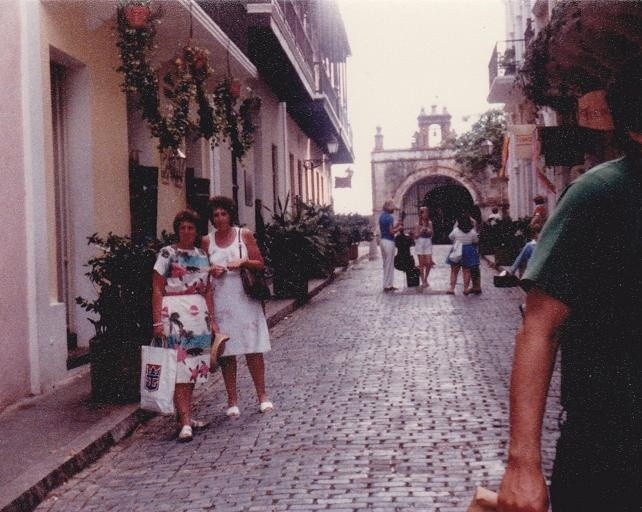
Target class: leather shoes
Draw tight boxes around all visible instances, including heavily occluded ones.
[178,418,209,439]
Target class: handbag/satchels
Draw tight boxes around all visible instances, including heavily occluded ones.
[449,240,463,264]
[140,336,177,414]
[241,264,270,299]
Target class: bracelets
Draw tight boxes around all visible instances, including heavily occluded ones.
[152,323,163,326]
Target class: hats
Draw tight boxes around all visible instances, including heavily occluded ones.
[383,201,401,210]
[211,333,230,367]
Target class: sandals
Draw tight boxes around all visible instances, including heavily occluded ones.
[260,402,272,412]
[226,407,240,417]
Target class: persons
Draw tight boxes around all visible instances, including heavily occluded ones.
[152,209,220,442]
[528,193,549,228]
[509,221,542,317]
[493,59,640,511]
[200,196,273,418]
[378,199,510,294]
[570,164,589,181]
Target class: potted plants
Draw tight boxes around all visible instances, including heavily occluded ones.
[75,229,179,406]
[115,1,262,158]
[251,193,370,304]
